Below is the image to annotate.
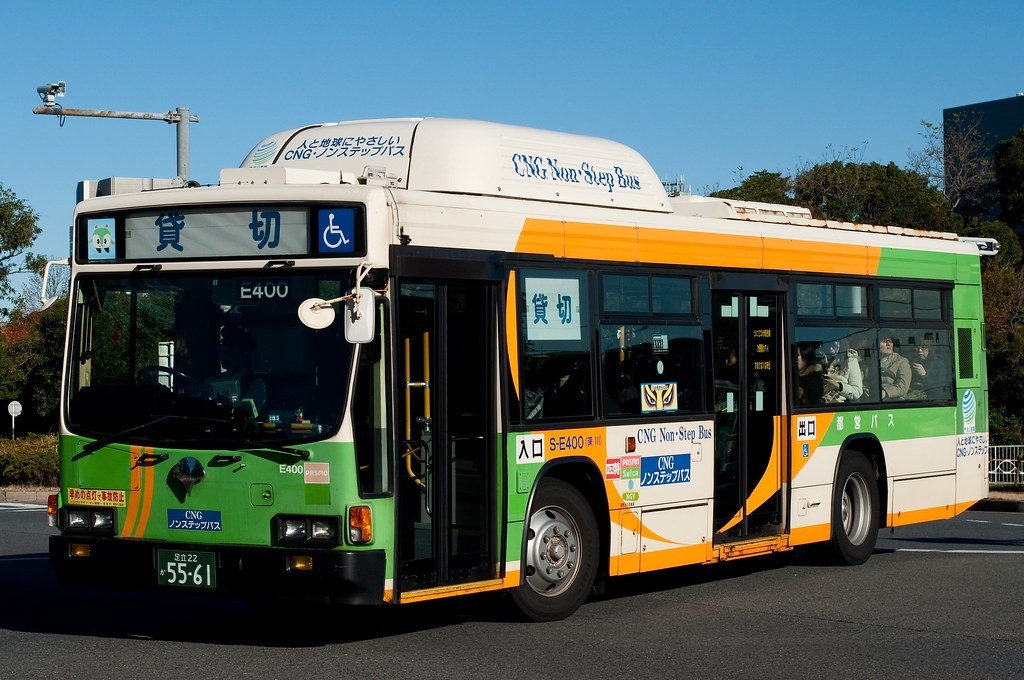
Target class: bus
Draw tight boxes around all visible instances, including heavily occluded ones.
[39,120,988,620]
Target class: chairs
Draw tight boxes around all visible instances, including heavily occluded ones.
[80,271,956,444]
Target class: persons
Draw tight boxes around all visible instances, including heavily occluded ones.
[878,331,911,402]
[798,341,823,404]
[910,333,945,401]
[722,349,738,383]
[822,334,863,403]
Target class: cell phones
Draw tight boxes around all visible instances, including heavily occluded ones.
[823,376,830,379]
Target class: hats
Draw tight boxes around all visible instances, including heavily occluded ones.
[879,329,894,343]
[221,323,259,351]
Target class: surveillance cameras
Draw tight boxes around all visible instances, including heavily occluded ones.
[37,81,66,97]
